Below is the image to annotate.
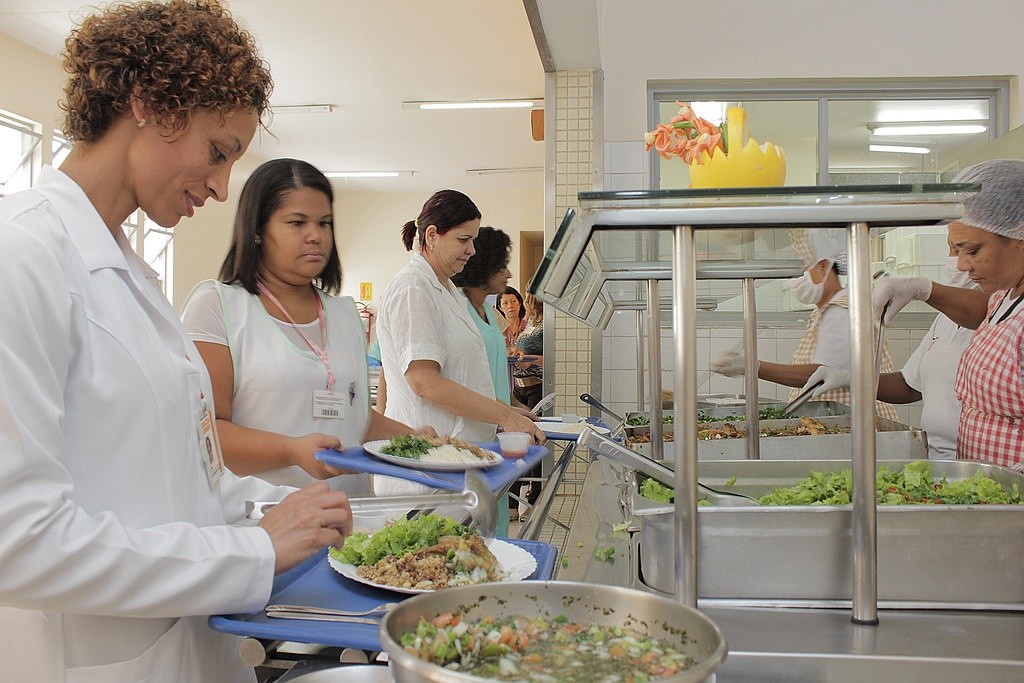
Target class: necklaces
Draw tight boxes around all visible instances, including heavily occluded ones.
[988,287,1024,328]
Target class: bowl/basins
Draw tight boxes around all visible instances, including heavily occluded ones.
[381,580,729,682]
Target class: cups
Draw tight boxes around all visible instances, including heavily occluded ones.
[561,413,580,423]
[496,432,533,459]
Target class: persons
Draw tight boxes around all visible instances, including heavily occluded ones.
[495,286,543,524]
[204,437,215,463]
[713,258,899,428]
[450,227,541,412]
[0,1,353,683]
[800,229,988,461]
[373,190,546,498]
[872,160,1024,477]
[177,159,440,499]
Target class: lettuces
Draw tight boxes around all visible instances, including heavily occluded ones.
[328,513,480,568]
[637,462,1024,512]
[560,541,624,566]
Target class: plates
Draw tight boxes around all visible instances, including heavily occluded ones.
[363,439,505,472]
[327,531,537,592]
[536,416,588,420]
[533,422,611,434]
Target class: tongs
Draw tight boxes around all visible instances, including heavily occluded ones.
[576,426,763,507]
[778,380,824,417]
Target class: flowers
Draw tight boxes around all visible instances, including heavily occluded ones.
[642,99,730,166]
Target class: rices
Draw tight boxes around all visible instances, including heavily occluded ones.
[416,444,489,464]
[447,549,488,584]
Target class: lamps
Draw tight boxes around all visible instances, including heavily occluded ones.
[321,170,417,177]
[402,98,544,111]
[865,119,991,155]
[266,104,332,113]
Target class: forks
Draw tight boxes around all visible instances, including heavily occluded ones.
[265,602,399,616]
[533,399,555,416]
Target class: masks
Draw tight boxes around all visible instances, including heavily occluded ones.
[785,256,834,306]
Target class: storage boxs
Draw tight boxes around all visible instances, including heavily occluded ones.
[883,225,952,311]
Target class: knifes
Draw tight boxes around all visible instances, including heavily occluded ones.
[531,392,557,413]
[265,611,382,627]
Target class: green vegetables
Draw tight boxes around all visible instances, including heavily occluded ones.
[380,434,438,460]
[630,407,840,433]
[396,615,692,683]
